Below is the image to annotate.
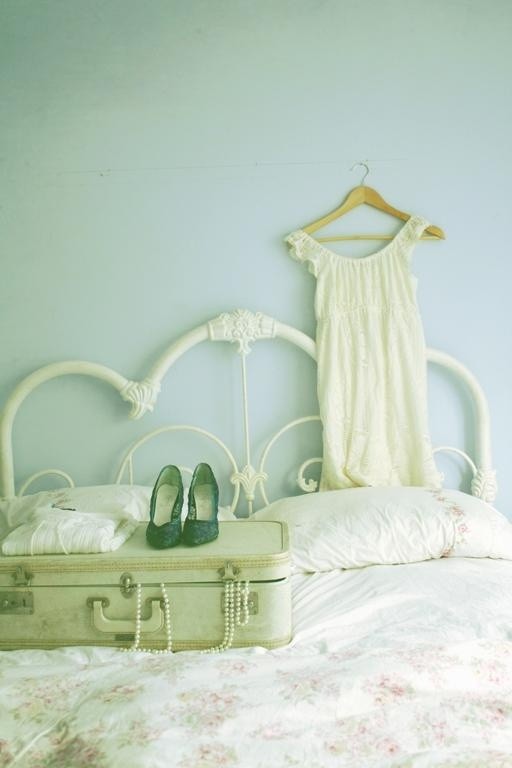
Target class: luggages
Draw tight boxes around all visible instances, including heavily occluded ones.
[1,518,294,648]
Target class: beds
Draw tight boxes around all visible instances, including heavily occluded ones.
[1,309,511,768]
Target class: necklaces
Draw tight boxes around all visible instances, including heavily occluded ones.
[117,583,172,654]
[199,580,234,653]
[235,580,249,627]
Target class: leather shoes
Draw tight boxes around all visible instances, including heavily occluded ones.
[182,462,220,548]
[145,464,185,549]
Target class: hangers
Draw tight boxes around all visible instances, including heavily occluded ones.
[287,163,445,244]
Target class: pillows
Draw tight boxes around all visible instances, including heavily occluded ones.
[243,485,511,574]
[6,484,240,528]
